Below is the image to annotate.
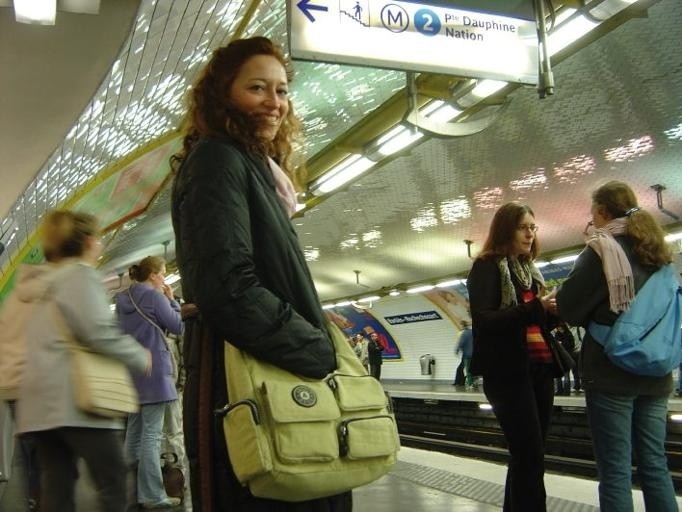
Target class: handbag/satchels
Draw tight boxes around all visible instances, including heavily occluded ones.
[66,346,140,417]
[214,321,401,503]
[603,263,681,378]
[167,338,178,382]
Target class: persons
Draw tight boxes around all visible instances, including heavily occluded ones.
[324,308,353,330]
[466,199,561,511]
[348,337,362,358]
[167,36,354,510]
[366,331,384,381]
[0,210,153,512]
[455,320,479,391]
[114,254,189,511]
[356,332,370,374]
[552,176,681,512]
[436,289,471,326]
[549,323,589,396]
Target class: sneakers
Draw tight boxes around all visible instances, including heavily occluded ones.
[143,497,180,507]
